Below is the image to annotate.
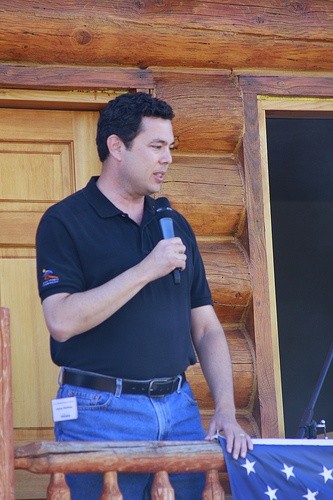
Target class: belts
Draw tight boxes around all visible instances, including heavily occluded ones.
[60,368,186,397]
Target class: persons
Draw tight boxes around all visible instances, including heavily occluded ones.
[35,91,253,500]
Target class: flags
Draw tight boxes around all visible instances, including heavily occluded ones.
[213,433,333,500]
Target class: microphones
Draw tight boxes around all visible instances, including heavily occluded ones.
[155,197,181,286]
